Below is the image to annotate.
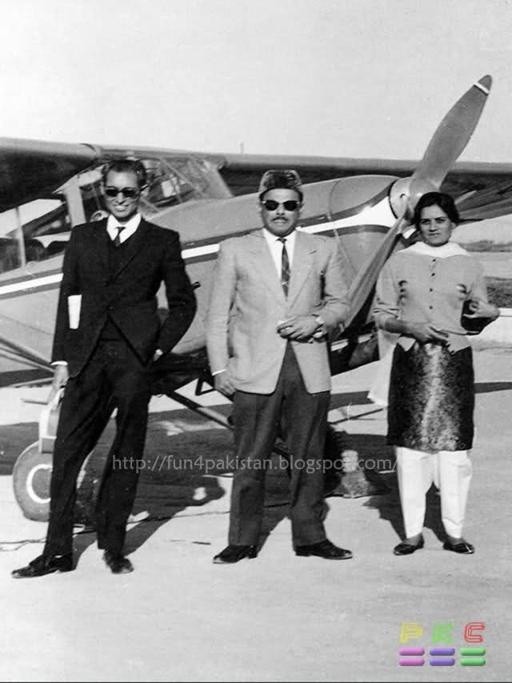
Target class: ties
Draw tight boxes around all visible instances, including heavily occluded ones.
[112,226,124,249]
[277,235,292,301]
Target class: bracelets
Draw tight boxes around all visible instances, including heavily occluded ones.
[314,315,324,325]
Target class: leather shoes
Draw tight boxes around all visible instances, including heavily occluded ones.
[12,551,76,578]
[393,536,425,556]
[294,538,352,560]
[103,548,134,575]
[213,543,257,564]
[444,538,474,555]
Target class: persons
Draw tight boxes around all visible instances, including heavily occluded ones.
[204,168,359,564]
[367,189,500,558]
[9,156,199,580]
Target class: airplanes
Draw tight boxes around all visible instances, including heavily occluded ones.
[0,72,510,533]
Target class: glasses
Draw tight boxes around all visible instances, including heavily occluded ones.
[105,187,142,197]
[260,199,302,210]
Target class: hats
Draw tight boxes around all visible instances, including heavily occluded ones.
[258,169,304,201]
